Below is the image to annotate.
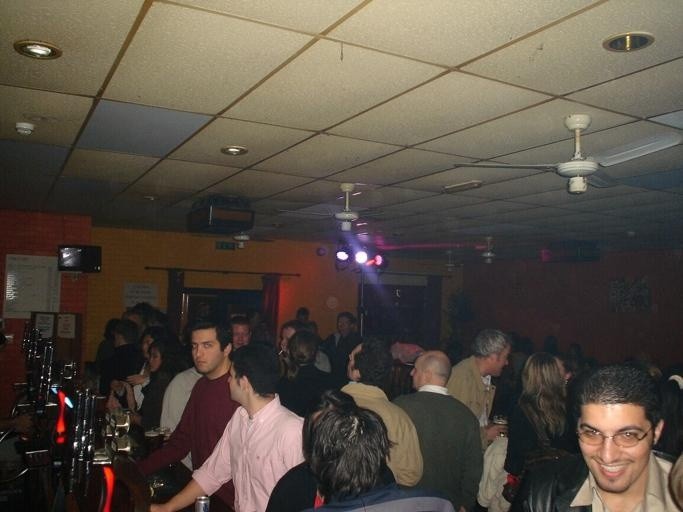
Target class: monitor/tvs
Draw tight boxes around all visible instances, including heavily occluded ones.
[58,244,102,272]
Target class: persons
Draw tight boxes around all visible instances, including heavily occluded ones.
[0,412,34,463]
[84,299,682,512]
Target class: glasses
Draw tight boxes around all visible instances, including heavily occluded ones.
[576,422,655,447]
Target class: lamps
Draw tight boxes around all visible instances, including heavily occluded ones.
[333,237,390,275]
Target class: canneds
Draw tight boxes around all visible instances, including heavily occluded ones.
[195,496,210,512]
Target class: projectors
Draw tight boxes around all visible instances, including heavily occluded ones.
[186,190,255,234]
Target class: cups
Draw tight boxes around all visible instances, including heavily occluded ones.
[492,413,508,439]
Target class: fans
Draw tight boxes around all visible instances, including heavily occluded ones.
[453,113,682,196]
[480,236,496,264]
[274,183,385,234]
[233,231,274,250]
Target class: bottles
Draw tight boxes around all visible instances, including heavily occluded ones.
[143,425,173,457]
